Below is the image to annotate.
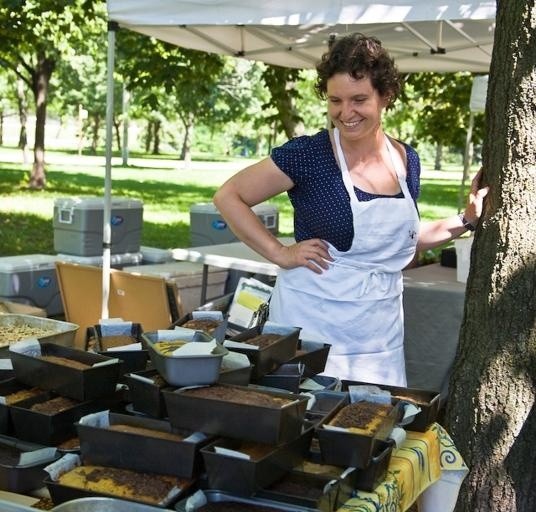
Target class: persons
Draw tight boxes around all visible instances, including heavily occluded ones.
[213,32,490,388]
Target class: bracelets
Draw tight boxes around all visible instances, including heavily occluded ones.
[459,214,474,231]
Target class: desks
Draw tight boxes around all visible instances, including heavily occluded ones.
[172,234,296,308]
[401,261,468,395]
[0,422,469,511]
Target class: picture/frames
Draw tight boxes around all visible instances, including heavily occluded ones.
[53,260,171,347]
[165,280,185,322]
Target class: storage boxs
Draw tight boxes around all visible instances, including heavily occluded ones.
[189,201,280,247]
[55,253,142,270]
[225,268,271,295]
[138,244,173,266]
[0,253,65,317]
[53,198,143,256]
[124,262,228,315]
